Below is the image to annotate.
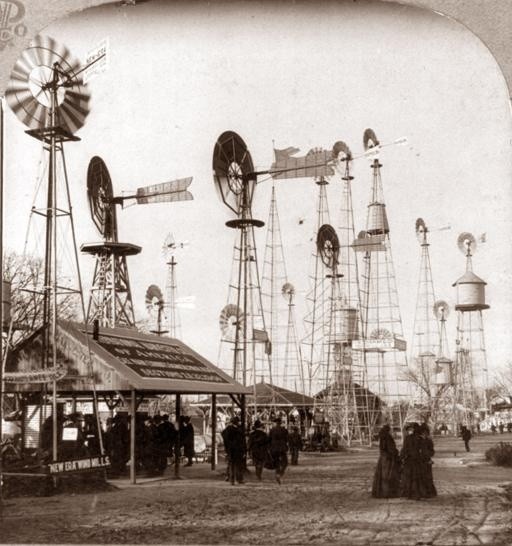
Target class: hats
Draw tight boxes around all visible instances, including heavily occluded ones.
[230,418,239,424]
[254,421,264,428]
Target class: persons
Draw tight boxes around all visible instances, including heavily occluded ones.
[41,411,194,476]
[499,422,504,433]
[440,422,448,435]
[462,425,471,451]
[491,423,496,432]
[372,420,437,500]
[221,417,301,484]
